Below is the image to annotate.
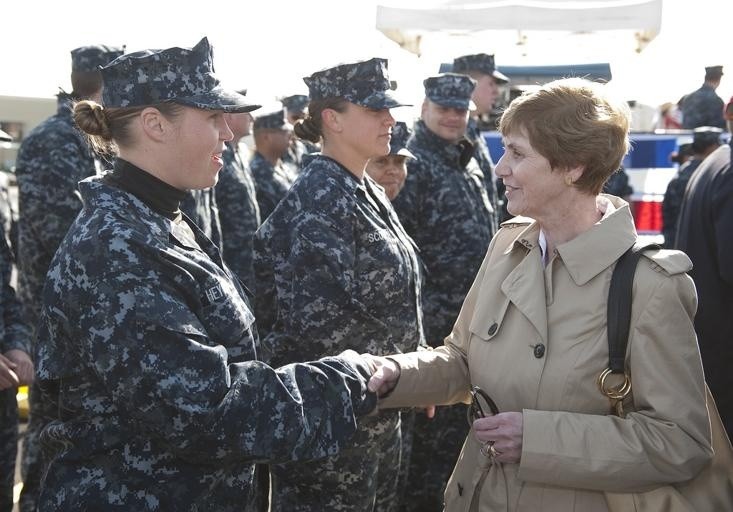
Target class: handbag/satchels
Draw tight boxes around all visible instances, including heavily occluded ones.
[597,244,733,512]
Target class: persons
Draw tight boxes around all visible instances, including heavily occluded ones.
[666,143,695,166]
[450,52,509,229]
[681,65,726,129]
[360,79,713,512]
[203,89,262,288]
[674,96,733,433]
[252,57,436,512]
[248,110,294,227]
[365,121,415,200]
[276,92,322,160]
[11,44,126,331]
[0,128,36,512]
[397,74,498,507]
[659,126,724,251]
[18,37,384,511]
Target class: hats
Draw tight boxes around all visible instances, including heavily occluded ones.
[280,94,310,109]
[72,44,124,74]
[250,110,286,128]
[387,122,418,160]
[454,53,509,84]
[211,87,262,112]
[693,125,724,143]
[97,36,263,115]
[423,72,477,111]
[705,65,724,78]
[303,57,415,110]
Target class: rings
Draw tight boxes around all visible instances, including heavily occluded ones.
[479,440,501,460]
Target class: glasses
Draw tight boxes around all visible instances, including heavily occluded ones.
[468,383,501,433]
